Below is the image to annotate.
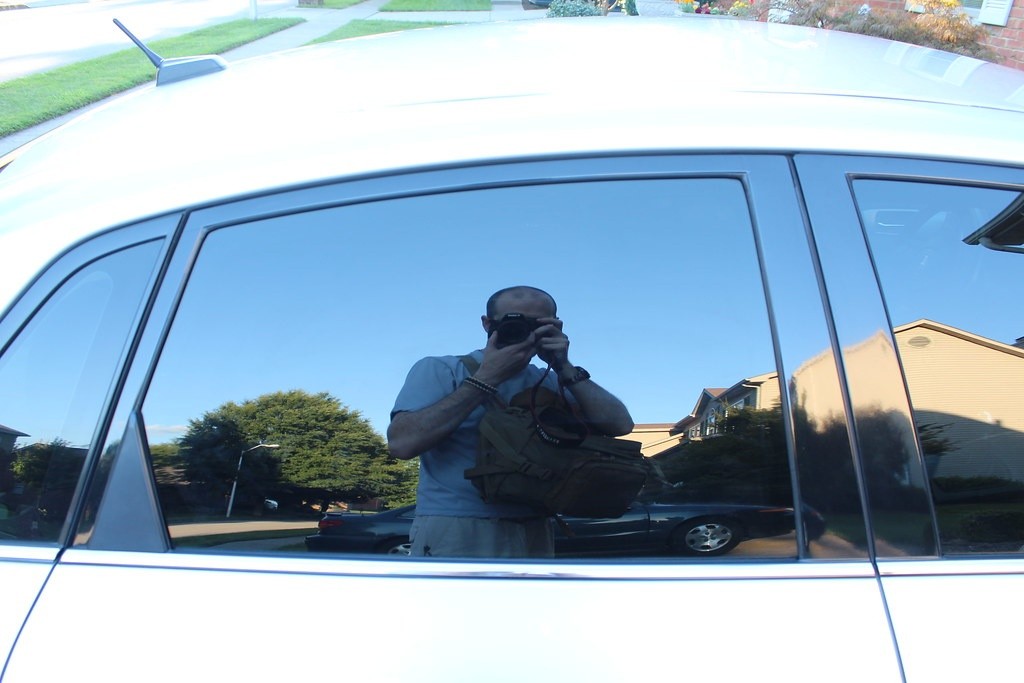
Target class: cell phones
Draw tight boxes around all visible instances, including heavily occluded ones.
[497,312,542,350]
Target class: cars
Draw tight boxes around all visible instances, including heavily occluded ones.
[0,18,1024,683]
[307,470,798,557]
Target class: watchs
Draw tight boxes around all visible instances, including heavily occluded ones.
[559,366,589,387]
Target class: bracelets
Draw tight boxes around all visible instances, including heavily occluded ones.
[463,375,497,396]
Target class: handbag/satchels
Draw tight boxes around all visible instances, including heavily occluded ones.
[456,351,645,519]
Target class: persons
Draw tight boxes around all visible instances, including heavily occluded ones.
[387,286,636,559]
